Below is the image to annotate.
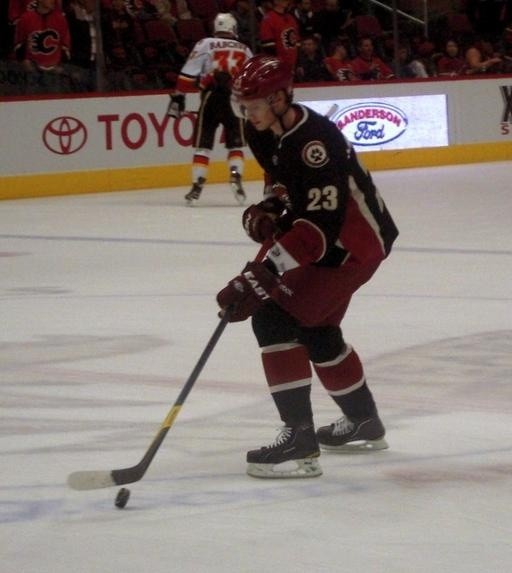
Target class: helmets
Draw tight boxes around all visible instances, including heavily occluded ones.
[214,12,240,39]
[230,54,294,132]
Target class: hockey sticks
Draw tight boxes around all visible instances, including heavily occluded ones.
[67,236,274,492]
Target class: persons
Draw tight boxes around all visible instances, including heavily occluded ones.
[216,56,398,465]
[170,13,255,201]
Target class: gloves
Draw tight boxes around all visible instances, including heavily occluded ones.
[241,194,294,243]
[216,260,282,322]
[168,93,188,118]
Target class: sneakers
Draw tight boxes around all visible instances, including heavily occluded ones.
[316,407,386,447]
[184,177,207,201]
[246,418,321,464]
[229,173,247,196]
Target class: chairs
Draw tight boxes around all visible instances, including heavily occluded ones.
[2,1,512,95]
